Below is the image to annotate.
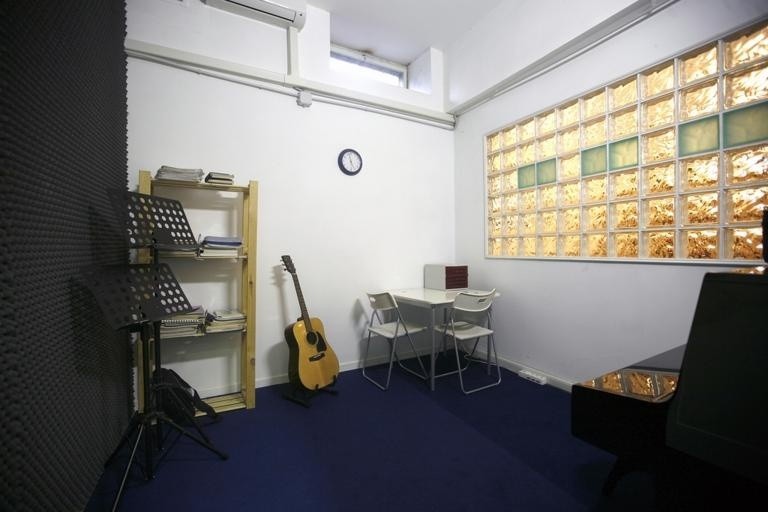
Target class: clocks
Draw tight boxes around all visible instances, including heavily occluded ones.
[338,149,363,176]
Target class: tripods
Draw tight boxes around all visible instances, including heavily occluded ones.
[113,339,227,512]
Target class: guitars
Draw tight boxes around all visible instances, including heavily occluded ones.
[280,256,339,394]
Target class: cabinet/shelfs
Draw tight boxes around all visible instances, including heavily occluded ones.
[136,168,259,420]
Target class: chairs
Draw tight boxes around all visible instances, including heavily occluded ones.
[360,288,502,394]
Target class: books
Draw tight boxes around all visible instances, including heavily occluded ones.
[153,164,235,185]
[148,305,245,339]
[155,236,244,257]
